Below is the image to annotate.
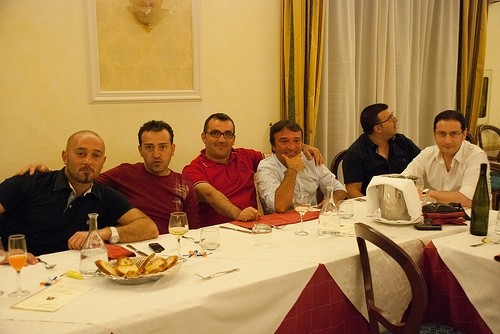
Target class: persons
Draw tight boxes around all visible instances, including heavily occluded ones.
[16,120,198,236]
[181,113,326,229]
[257,120,347,213]
[342,103,421,200]
[401,110,492,211]
[0,130,159,266]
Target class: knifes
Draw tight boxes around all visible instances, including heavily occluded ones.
[219,226,252,234]
[126,244,149,256]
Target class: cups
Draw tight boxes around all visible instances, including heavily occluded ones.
[337,200,354,220]
[199,227,221,251]
[420,198,438,215]
[252,223,272,244]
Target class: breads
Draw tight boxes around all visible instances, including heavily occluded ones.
[94,253,179,277]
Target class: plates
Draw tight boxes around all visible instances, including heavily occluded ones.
[374,215,425,225]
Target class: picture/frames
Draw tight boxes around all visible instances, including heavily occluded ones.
[85,0,202,102]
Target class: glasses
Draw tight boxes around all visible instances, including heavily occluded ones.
[374,111,394,125]
[434,131,462,138]
[205,130,235,139]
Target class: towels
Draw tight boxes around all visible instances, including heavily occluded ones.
[363,175,423,223]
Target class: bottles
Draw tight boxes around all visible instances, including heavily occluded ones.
[79,213,109,277]
[469,162,490,236]
[317,185,341,238]
[495,211,500,234]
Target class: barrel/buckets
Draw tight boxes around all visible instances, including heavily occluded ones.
[377,173,420,220]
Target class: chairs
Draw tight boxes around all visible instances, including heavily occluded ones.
[355,223,460,334]
[466,124,500,211]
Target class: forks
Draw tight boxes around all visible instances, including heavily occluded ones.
[36,258,57,269]
[270,224,286,229]
[182,235,205,243]
[195,268,240,280]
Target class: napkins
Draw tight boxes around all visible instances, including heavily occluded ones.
[230,210,320,229]
[423,211,466,224]
[105,244,136,260]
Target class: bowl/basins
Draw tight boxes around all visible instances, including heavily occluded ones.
[94,257,187,284]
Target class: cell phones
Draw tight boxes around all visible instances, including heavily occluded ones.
[149,243,165,253]
[414,223,443,230]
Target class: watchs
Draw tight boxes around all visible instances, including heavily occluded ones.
[422,188,429,194]
[107,226,119,244]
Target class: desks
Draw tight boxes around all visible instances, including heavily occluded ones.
[432,224,500,334]
[0,196,500,334]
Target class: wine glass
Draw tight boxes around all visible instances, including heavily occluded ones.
[168,211,189,263]
[293,191,310,236]
[0,235,6,296]
[8,234,30,298]
